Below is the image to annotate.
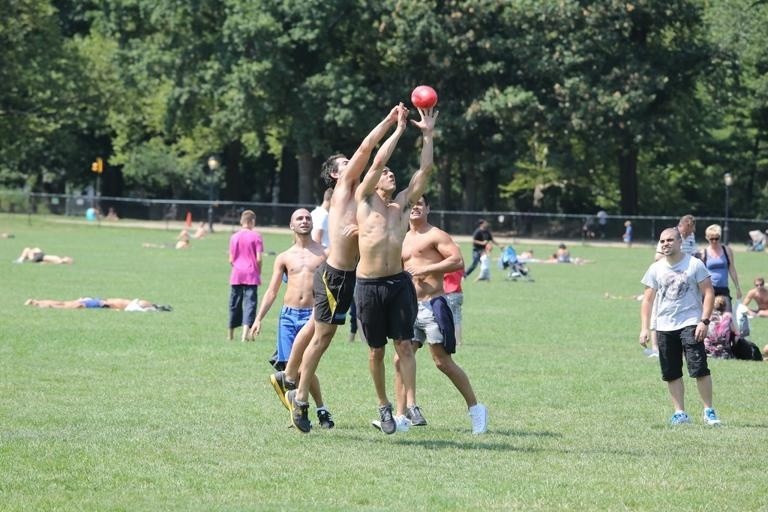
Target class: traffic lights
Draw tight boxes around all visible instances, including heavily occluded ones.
[90,157,102,174]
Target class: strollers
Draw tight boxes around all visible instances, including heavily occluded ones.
[497,246,534,283]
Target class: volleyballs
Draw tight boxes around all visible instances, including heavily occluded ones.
[411,86,437,109]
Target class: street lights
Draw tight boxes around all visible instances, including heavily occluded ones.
[722,173,734,245]
[205,155,220,231]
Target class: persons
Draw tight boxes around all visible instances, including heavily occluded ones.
[518,250,533,259]
[557,244,568,263]
[270,103,409,433]
[17,247,73,265]
[349,302,359,342]
[477,243,493,280]
[465,219,500,276]
[623,220,633,247]
[310,189,334,246]
[250,208,335,431]
[144,240,189,249]
[228,210,264,343]
[25,297,153,309]
[372,193,487,435]
[443,270,465,346]
[106,207,118,221]
[597,209,608,238]
[86,205,103,221]
[178,221,206,239]
[639,214,768,427]
[354,104,439,435]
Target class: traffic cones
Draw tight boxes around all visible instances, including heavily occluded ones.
[185,211,193,228]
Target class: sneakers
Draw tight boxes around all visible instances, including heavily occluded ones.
[372,403,426,434]
[468,401,487,434]
[704,408,721,427]
[270,369,334,432]
[671,410,688,425]
